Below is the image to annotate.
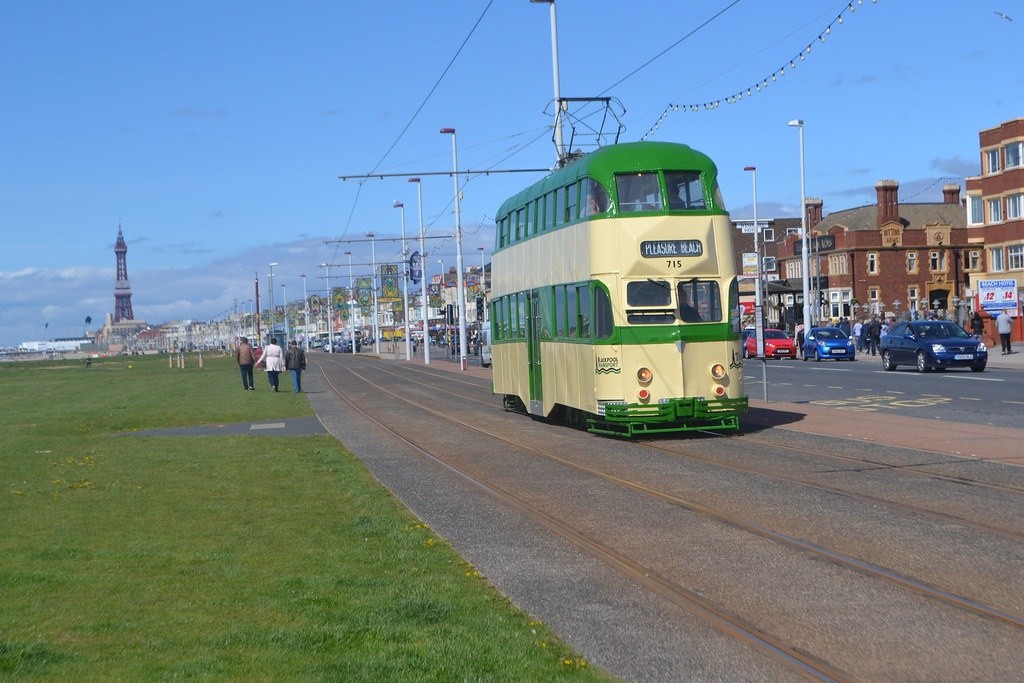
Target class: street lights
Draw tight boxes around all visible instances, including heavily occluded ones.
[476,247,486,322]
[743,166,765,360]
[321,263,333,354]
[440,127,468,372]
[437,259,446,289]
[300,274,309,354]
[393,202,411,361]
[366,232,380,354]
[408,176,431,364]
[281,284,288,351]
[267,263,278,345]
[787,119,812,343]
[344,251,356,355]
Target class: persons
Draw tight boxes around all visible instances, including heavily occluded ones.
[86,356,91,368]
[585,191,601,215]
[467,332,477,355]
[970,312,984,340]
[237,338,306,393]
[826,317,850,338]
[679,289,702,322]
[996,309,1013,355]
[933,314,938,321]
[670,186,686,209]
[853,317,897,356]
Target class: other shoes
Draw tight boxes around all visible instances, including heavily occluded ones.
[864,348,868,354]
[271,385,276,392]
[1007,350,1012,354]
[250,386,254,390]
[1001,351,1005,355]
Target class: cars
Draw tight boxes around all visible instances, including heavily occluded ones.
[409,325,472,355]
[878,320,988,374]
[310,327,373,354]
[800,326,855,362]
[744,328,796,360]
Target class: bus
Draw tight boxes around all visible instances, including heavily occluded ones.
[491,93,752,443]
